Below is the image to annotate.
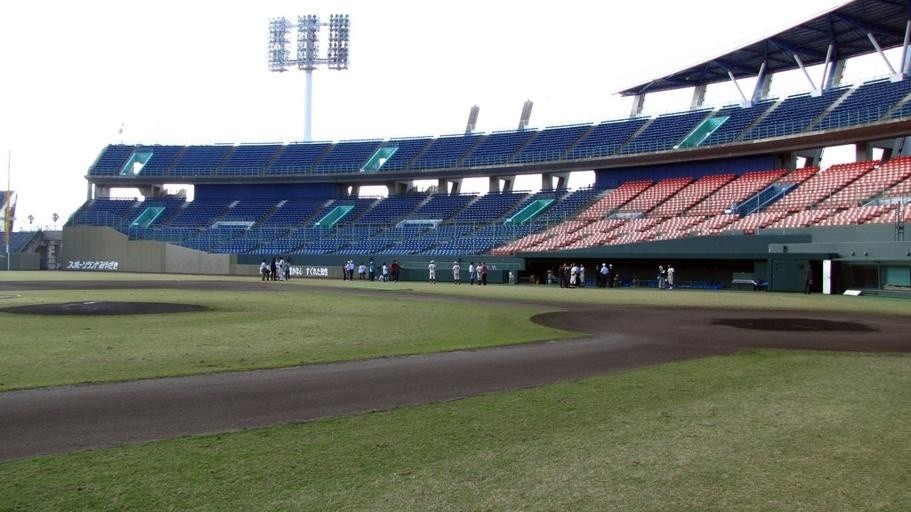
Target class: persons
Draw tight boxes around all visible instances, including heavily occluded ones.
[260,257,291,281]
[803,264,813,293]
[559,262,585,288]
[452,261,460,285]
[599,263,615,288]
[469,261,488,285]
[428,260,436,283]
[657,265,674,289]
[343,260,400,282]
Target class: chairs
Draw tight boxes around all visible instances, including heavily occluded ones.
[344,278,399,281]
[559,285,585,288]
[471,282,487,285]
[261,278,290,281]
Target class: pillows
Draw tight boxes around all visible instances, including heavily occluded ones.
[347,259,354,264]
[430,260,435,262]
[602,263,613,266]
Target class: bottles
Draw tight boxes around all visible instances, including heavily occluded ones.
[454,261,459,263]
